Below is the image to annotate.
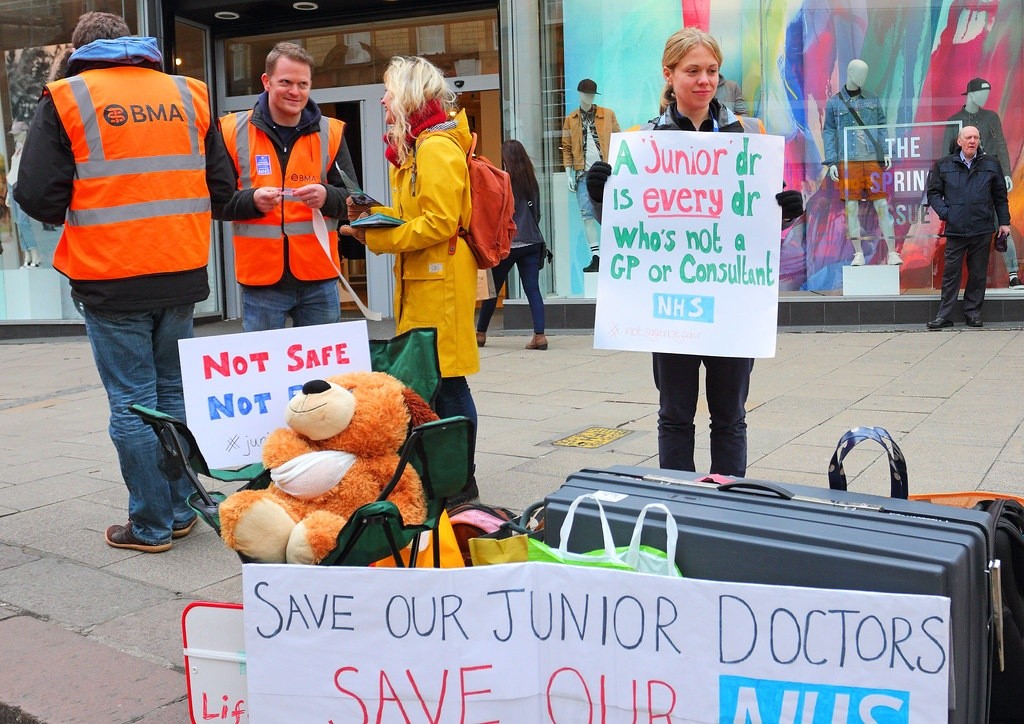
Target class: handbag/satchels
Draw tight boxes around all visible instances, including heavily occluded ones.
[995,232,1007,253]
[875,142,886,167]
[444,501,683,577]
[829,426,1024,509]
[539,243,547,270]
[477,268,498,300]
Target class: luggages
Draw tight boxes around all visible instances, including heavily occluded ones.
[518,464,995,723]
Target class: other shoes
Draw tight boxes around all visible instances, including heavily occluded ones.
[445,481,479,510]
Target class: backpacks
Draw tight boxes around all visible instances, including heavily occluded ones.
[976,496,1024,724]
[415,131,517,269]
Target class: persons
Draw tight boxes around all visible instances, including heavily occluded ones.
[562,80,621,271]
[475,139,554,350]
[926,126,1012,328]
[4,121,42,269]
[942,77,1024,290]
[338,52,483,513]
[585,26,805,478]
[822,58,904,266]
[714,73,748,117]
[10,10,237,553]
[209,42,360,332]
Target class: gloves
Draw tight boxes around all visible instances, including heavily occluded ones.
[586,161,612,203]
[339,197,372,246]
[776,190,803,220]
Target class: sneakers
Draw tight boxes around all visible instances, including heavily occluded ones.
[583,255,599,272]
[104,517,172,553]
[1009,277,1024,289]
[887,252,904,264]
[851,252,865,265]
[172,513,198,538]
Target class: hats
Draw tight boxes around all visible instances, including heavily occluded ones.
[961,77,991,95]
[577,79,601,95]
[7,122,29,136]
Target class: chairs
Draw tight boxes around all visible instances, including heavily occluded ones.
[125,327,481,568]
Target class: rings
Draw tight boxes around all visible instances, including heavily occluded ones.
[307,200,310,204]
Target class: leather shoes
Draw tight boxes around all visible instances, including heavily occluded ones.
[966,318,983,326]
[926,318,953,328]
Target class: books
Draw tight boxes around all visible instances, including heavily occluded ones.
[350,213,406,228]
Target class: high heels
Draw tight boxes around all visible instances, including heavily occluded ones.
[526,334,548,349]
[476,331,486,347]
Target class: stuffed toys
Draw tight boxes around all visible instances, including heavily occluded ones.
[218,371,444,564]
